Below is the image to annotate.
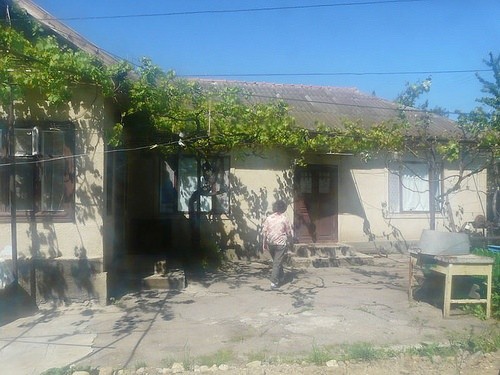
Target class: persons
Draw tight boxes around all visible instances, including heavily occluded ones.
[261,199,293,288]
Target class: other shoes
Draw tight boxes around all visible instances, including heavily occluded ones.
[270,276,284,288]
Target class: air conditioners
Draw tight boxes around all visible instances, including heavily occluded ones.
[0,126,65,158]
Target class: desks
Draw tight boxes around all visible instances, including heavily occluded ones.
[407,247,494,320]
